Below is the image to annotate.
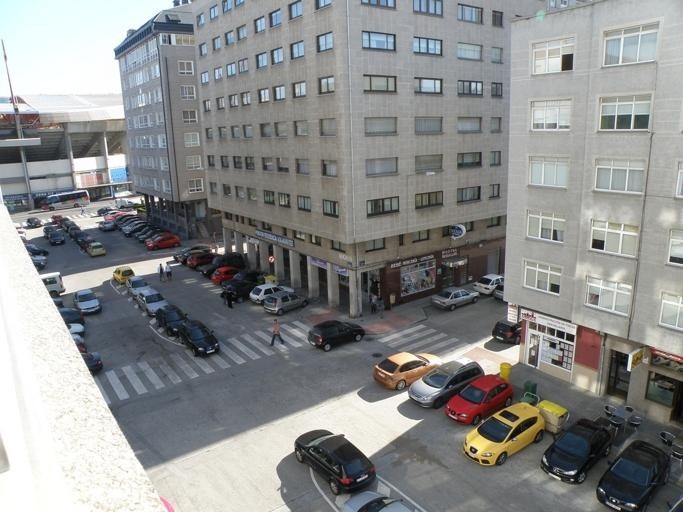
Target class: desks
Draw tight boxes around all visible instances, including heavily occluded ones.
[672,438,683,454]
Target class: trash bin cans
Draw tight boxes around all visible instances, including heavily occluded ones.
[524,380,537,398]
[499,362,513,380]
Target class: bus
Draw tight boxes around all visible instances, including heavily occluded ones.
[45,188,92,211]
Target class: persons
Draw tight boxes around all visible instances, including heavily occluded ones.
[158,264,166,282]
[222,289,227,304]
[226,289,234,309]
[269,319,284,346]
[81,205,85,215]
[165,262,172,280]
[369,292,378,313]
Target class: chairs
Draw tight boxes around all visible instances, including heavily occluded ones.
[660,431,675,446]
[604,405,641,436]
[671,450,683,468]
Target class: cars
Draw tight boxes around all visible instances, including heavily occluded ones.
[334,491,415,512]
[291,427,377,496]
[171,244,308,316]
[39,270,102,376]
[12,213,107,269]
[428,272,510,311]
[112,265,220,360]
[304,321,366,350]
[95,197,183,253]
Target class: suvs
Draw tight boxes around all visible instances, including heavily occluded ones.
[489,316,525,348]
